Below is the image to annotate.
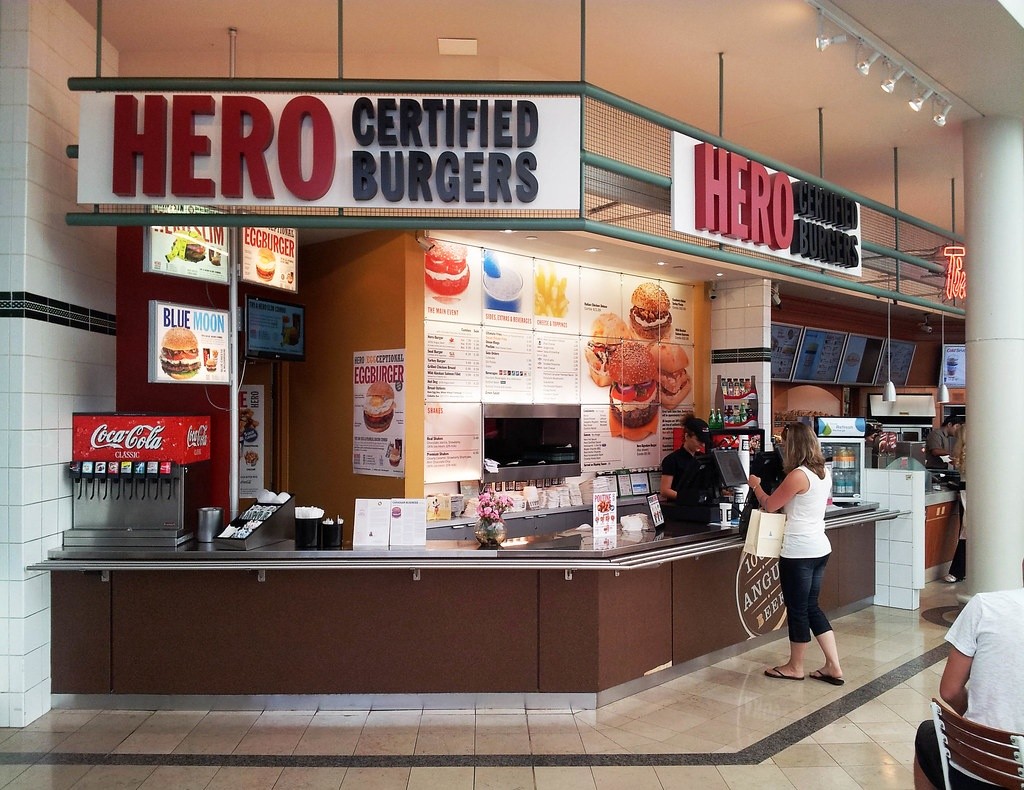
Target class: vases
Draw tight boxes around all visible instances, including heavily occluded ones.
[473,516,505,550]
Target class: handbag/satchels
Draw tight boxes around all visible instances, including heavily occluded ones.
[743,495,786,558]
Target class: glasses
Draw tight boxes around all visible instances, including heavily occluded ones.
[785,424,790,435]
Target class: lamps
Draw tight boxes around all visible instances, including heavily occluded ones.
[883,298,897,401]
[918,316,932,333]
[418,230,436,250]
[937,313,949,403]
[933,105,952,124]
[815,35,848,50]
[858,52,879,75]
[881,67,905,92]
[909,90,934,111]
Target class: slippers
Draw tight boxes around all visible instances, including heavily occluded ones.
[764,666,804,680]
[809,670,844,685]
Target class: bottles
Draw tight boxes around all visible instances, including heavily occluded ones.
[715,409,724,428]
[821,445,855,493]
[724,402,754,424]
[721,377,752,396]
[708,409,715,428]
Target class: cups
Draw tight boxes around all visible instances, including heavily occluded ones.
[802,343,819,367]
[947,358,958,378]
[295,517,322,548]
[321,522,343,549]
[719,503,732,524]
[194,507,223,543]
[733,435,750,517]
[483,267,524,312]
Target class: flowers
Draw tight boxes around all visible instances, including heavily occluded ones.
[475,487,516,524]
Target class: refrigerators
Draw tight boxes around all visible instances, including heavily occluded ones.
[811,415,866,506]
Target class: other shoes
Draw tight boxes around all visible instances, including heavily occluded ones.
[944,574,963,583]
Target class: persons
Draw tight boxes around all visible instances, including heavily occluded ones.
[913,561,1024,789]
[925,416,966,583]
[748,422,845,686]
[659,413,715,504]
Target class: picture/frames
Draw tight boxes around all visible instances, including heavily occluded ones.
[770,322,803,382]
[792,327,848,384]
[837,332,886,385]
[938,343,966,388]
[873,339,917,386]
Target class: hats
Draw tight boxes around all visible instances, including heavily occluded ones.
[864,424,882,438]
[687,418,710,442]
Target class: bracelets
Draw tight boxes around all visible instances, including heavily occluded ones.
[751,484,761,492]
